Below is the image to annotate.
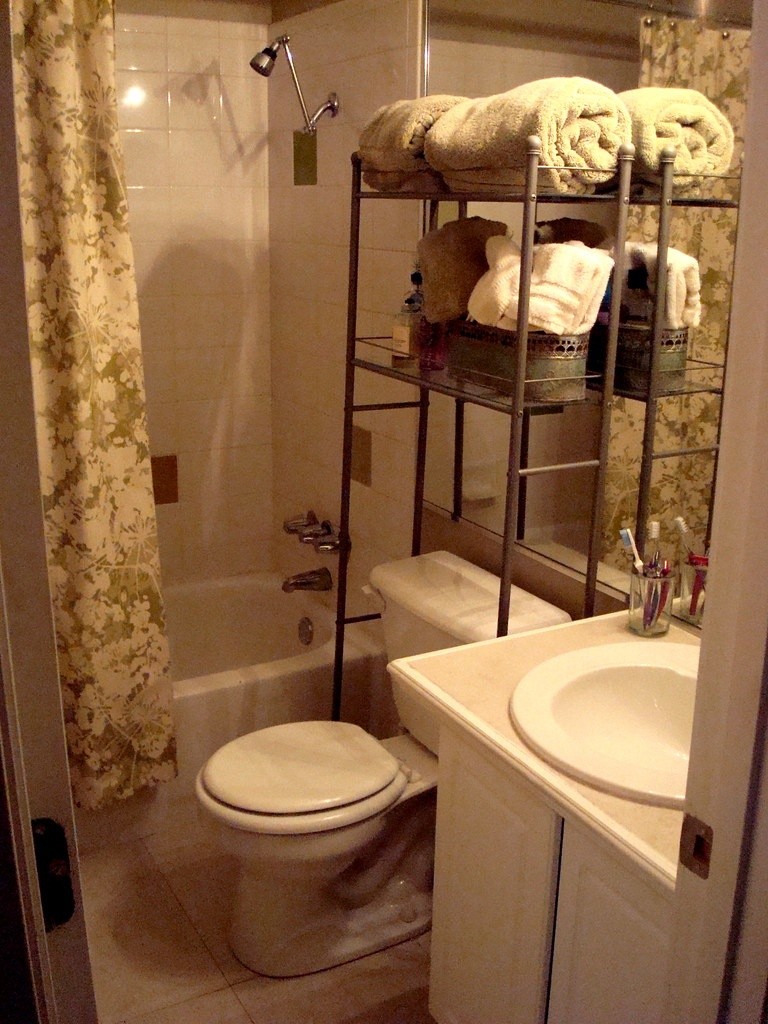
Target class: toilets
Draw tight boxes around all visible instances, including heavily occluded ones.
[196,548,573,980]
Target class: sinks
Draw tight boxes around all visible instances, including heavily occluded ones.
[509,640,701,811]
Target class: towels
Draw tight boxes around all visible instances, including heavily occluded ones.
[422,76,632,195]
[617,87,734,195]
[416,215,510,324]
[592,231,703,331]
[468,233,614,335]
[358,93,471,194]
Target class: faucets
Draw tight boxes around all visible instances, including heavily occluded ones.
[281,567,333,594]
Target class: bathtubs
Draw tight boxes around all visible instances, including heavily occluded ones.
[163,570,384,786]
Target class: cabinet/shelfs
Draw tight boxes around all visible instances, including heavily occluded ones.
[327,143,635,723]
[449,153,745,575]
[428,723,674,1024]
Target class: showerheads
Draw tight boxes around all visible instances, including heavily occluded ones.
[250,35,292,77]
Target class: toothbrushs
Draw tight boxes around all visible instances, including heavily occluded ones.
[619,528,644,576]
[649,521,661,568]
[674,516,696,566]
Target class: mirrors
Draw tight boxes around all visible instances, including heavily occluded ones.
[418,0,752,631]
